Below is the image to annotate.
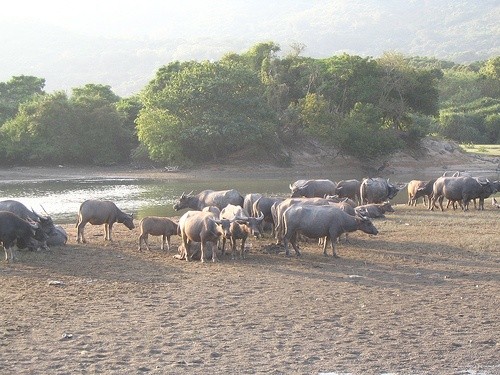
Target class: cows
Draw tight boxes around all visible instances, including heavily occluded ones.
[137,169,499,264]
[0,199,68,263]
[75,199,136,245]
